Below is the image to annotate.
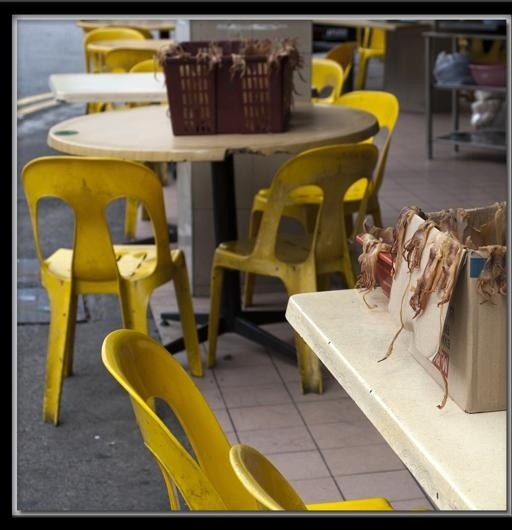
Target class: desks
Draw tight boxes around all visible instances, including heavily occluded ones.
[284,284,506,512]
[50,72,169,106]
[47,105,380,368]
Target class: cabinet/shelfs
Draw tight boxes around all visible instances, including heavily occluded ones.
[421,29,508,161]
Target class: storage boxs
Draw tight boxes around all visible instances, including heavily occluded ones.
[388,203,506,414]
[162,39,296,137]
[355,228,396,297]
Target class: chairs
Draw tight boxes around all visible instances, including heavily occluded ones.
[313,44,354,94]
[103,45,161,78]
[228,443,398,514]
[86,38,175,76]
[81,27,145,75]
[21,156,203,425]
[311,58,343,106]
[356,28,386,92]
[102,328,265,512]
[250,90,400,257]
[207,143,379,396]
